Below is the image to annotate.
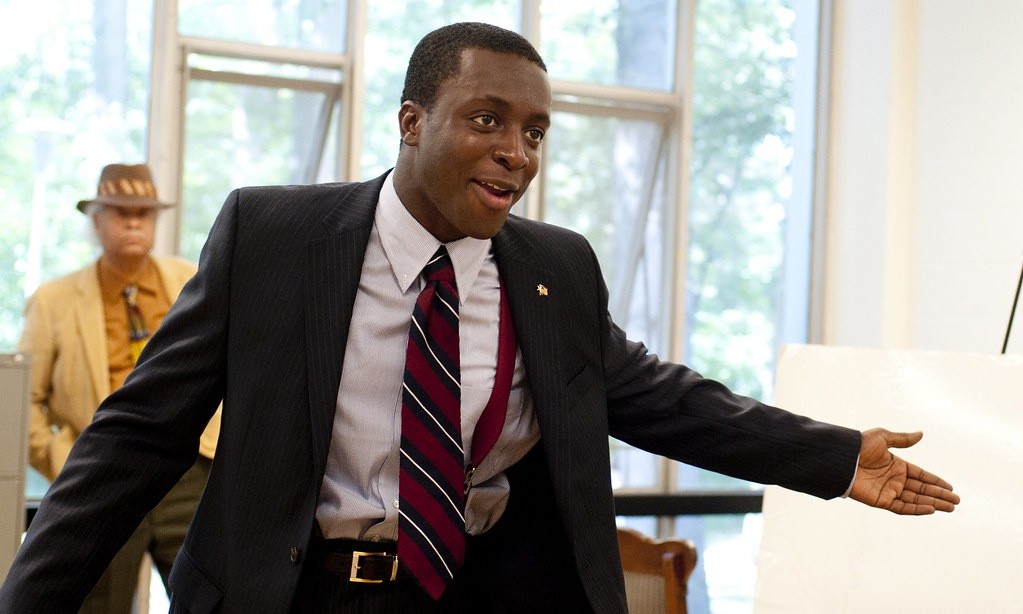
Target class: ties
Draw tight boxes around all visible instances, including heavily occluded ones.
[121,285,150,369]
[396,246,466,603]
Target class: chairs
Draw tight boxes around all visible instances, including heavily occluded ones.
[617,525,698,614]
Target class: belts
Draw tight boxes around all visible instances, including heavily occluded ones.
[310,549,399,585]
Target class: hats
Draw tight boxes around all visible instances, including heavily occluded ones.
[77,163,178,216]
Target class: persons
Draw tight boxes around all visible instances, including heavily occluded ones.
[18,160,223,614]
[0,20,960,614]
